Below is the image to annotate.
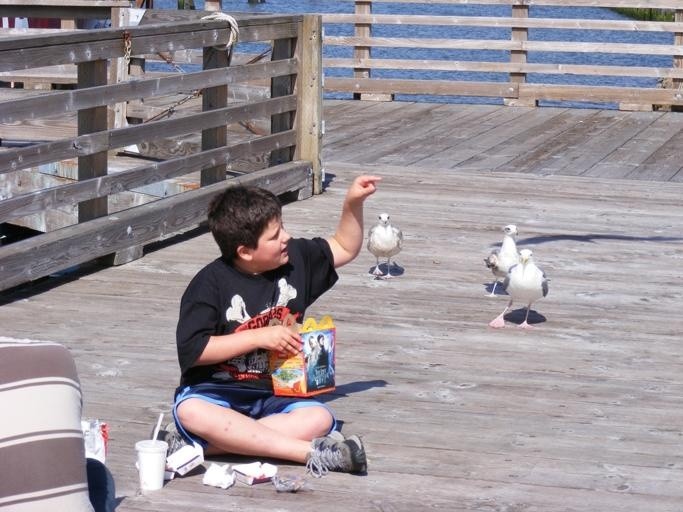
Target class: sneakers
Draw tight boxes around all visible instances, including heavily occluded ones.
[152,421,188,463]
[306,430,368,476]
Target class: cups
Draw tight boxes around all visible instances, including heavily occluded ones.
[135,439,167,493]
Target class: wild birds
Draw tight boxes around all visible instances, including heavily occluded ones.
[482,223,522,299]
[488,247,551,330]
[366,213,405,280]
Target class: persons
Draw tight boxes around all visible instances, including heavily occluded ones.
[309,336,330,379]
[172,174,382,475]
